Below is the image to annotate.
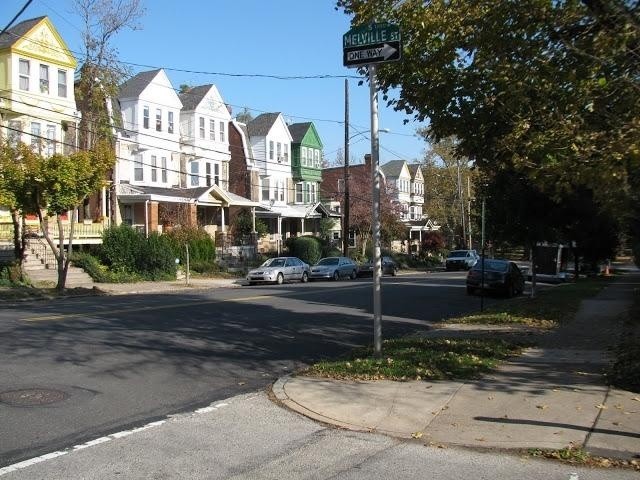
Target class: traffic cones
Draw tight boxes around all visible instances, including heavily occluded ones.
[604,266,609,275]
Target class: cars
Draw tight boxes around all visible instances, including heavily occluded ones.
[356,256,397,278]
[467,259,525,298]
[308,257,360,282]
[246,256,311,286]
[446,250,477,270]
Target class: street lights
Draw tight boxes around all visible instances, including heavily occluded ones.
[344,79,390,259]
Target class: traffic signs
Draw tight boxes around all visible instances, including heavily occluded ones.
[343,41,401,66]
[343,22,401,49]
